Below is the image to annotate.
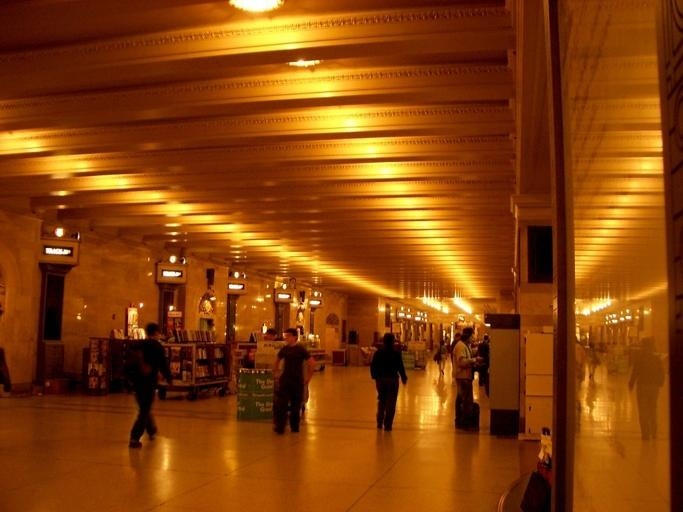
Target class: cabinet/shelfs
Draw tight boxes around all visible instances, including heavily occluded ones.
[158,340,230,400]
[518,330,554,441]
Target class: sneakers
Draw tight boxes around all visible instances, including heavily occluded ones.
[271,425,300,434]
[376,423,392,433]
[128,426,157,449]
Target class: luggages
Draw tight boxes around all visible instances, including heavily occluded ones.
[461,401,480,432]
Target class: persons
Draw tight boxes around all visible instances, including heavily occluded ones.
[272,328,316,434]
[437,328,489,431]
[370,333,407,431]
[123,323,172,447]
